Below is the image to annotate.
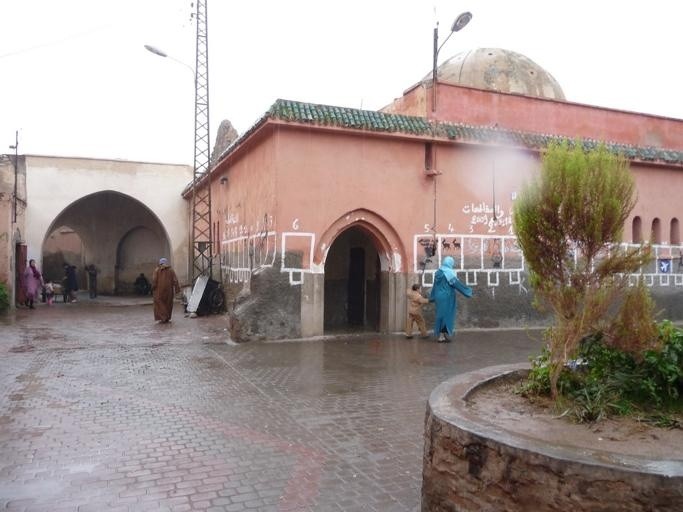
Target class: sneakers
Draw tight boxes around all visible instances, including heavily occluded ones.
[406,335,429,339]
[432,333,450,342]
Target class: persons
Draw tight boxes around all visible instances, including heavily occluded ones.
[24,260,44,309]
[427,255,475,343]
[62,264,78,302]
[16,259,28,307]
[152,258,180,324]
[42,271,48,302]
[135,272,150,296]
[84,264,100,298]
[43,279,54,305]
[405,283,432,340]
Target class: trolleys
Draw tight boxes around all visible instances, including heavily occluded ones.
[185,254,224,317]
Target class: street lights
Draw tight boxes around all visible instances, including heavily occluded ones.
[431,11,472,223]
[144,43,195,78]
